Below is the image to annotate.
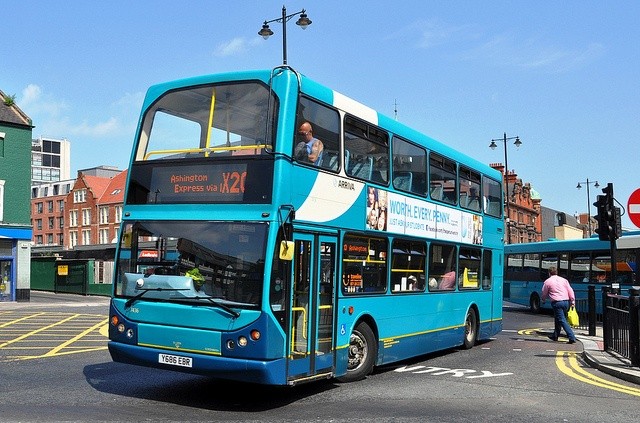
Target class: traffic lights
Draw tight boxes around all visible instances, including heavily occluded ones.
[593,195,608,240]
[604,208,622,240]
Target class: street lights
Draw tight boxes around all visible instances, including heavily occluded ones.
[576,178,599,238]
[258,5,312,65]
[489,132,521,244]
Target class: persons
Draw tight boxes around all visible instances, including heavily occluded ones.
[420,273,438,290]
[393,162,412,191]
[439,263,463,291]
[376,206,387,230]
[628,271,637,283]
[541,267,578,344]
[482,274,491,288]
[144,236,195,276]
[467,187,480,211]
[582,272,590,281]
[0,269,10,301]
[367,187,380,229]
[294,121,324,167]
[406,276,422,291]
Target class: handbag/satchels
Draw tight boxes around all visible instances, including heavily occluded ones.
[567,305,579,326]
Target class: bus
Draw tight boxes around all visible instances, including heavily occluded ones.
[503,231,640,313]
[108,66,504,385]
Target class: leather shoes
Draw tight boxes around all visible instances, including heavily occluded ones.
[566,339,576,343]
[548,335,558,340]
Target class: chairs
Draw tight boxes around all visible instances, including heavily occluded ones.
[490,197,501,217]
[468,196,481,212]
[363,286,384,292]
[318,308,333,332]
[348,158,373,180]
[394,172,412,191]
[577,275,585,283]
[443,187,456,204]
[429,183,443,200]
[459,191,469,209]
[322,150,349,174]
[486,198,491,215]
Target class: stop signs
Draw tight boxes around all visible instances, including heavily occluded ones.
[627,187,640,228]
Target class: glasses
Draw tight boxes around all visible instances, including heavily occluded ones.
[297,131,310,137]
